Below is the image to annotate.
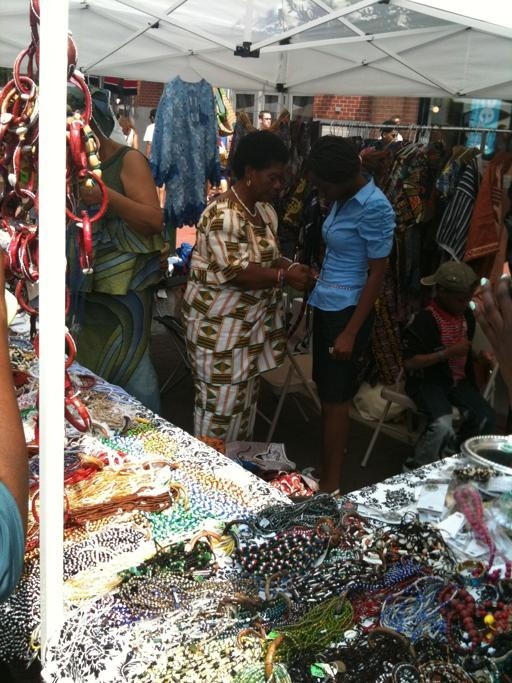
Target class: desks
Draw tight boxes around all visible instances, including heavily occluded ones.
[2,317,293,677]
[55,451,511,680]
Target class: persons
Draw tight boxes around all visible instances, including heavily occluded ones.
[1,245,32,606]
[0,85,512,506]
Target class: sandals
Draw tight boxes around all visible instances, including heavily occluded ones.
[303,466,343,501]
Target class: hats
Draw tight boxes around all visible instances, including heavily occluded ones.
[421,261,478,293]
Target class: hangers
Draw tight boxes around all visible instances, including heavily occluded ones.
[175,55,206,82]
[327,114,510,166]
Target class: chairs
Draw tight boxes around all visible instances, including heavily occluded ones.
[351,375,426,467]
[255,353,321,446]
[156,282,196,394]
